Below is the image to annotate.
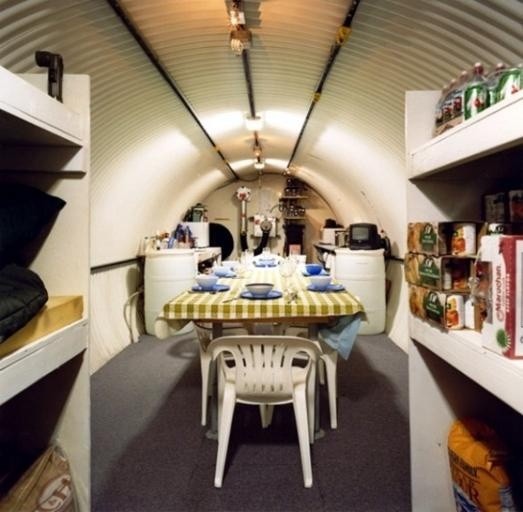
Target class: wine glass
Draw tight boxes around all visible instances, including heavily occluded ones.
[239,246,270,274]
[278,260,295,290]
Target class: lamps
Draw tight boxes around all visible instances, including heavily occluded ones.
[223,0,251,55]
[245,114,269,176]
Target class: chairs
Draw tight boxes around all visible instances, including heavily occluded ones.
[192,295,362,429]
[206,334,317,491]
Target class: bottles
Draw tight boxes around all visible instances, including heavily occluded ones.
[434,61,506,137]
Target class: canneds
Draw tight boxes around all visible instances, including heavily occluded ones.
[452,223,477,258]
[442,258,452,291]
[446,294,465,330]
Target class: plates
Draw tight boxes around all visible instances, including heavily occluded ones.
[240,291,282,299]
[215,272,236,277]
[302,272,329,277]
[306,284,344,292]
[191,285,230,292]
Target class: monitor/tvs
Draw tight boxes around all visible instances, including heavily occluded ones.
[350,224,381,250]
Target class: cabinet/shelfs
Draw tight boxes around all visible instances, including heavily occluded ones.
[280,194,308,221]
[0,65,90,512]
[315,242,386,337]
[402,82,523,512]
[141,246,222,336]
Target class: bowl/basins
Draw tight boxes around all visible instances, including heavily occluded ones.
[194,275,218,289]
[309,276,332,289]
[244,283,273,295]
[304,264,321,274]
